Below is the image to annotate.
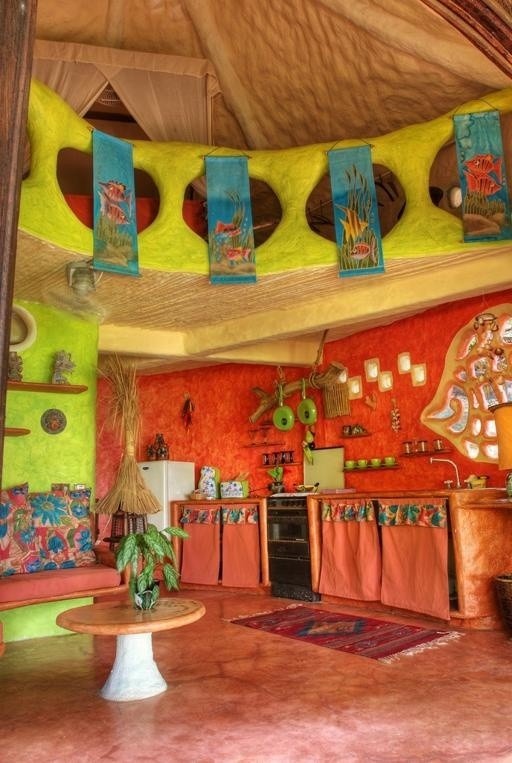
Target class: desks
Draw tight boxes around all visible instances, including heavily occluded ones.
[56,597,207,702]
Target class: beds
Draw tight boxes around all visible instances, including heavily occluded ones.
[22,39,223,241]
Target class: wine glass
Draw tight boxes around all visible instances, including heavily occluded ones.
[259,425,273,444]
[247,426,259,444]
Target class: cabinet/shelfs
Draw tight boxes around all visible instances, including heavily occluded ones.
[243,426,301,469]
[340,434,452,473]
[4,380,88,437]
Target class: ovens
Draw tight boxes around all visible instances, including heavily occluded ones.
[266,510,320,602]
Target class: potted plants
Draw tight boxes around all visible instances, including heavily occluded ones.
[114,523,191,610]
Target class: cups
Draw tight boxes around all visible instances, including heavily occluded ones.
[402,441,414,454]
[418,439,429,452]
[384,455,396,463]
[345,460,356,466]
[342,424,352,434]
[371,458,382,465]
[357,457,368,465]
[433,438,445,451]
[262,449,295,464]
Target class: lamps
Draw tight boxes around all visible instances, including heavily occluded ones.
[67,261,99,305]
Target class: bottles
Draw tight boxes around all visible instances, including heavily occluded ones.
[144,433,168,460]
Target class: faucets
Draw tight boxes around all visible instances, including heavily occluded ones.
[429,457,462,489]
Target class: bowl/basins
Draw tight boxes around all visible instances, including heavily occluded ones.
[292,481,319,492]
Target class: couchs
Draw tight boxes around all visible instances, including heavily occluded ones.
[0,491,134,657]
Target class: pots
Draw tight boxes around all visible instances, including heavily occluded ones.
[271,382,293,431]
[296,378,318,426]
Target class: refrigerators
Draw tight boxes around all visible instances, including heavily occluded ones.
[136,459,196,554]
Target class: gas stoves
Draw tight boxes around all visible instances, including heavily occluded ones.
[267,492,321,508]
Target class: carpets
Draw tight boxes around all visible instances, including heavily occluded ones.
[220,603,467,664]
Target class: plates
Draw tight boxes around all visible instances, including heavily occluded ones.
[356,465,370,467]
[368,464,382,467]
[343,465,356,469]
[382,462,399,466]
